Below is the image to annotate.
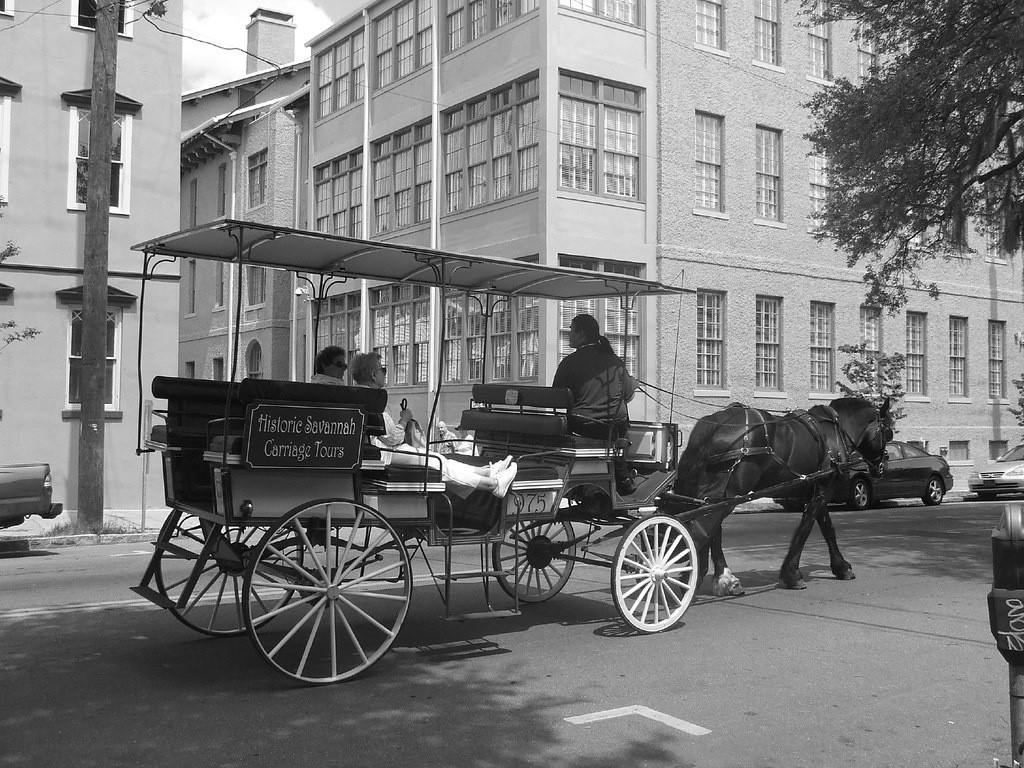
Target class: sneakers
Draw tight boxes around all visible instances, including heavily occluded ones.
[491,462,517,499]
[489,455,513,478]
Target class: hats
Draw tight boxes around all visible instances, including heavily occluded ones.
[455,426,466,430]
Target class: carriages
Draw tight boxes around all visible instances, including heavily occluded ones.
[130,219,894,687]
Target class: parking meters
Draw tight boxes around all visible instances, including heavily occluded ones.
[986,503,1024,768]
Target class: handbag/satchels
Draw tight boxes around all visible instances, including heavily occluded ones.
[400,398,426,449]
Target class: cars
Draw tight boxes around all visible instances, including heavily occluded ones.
[968,444,1024,501]
[772,440,954,511]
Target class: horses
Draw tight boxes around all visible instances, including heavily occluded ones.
[675,395,891,595]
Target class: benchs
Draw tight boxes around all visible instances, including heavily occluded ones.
[151,376,388,471]
[429,383,627,482]
[363,412,446,493]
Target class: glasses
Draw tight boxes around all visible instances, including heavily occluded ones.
[328,361,347,369]
[375,368,386,375]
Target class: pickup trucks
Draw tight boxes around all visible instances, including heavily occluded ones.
[0,462,63,530]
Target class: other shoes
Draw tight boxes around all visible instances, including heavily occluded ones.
[617,477,636,495]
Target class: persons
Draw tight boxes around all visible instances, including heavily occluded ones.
[308,345,518,500]
[550,314,640,497]
